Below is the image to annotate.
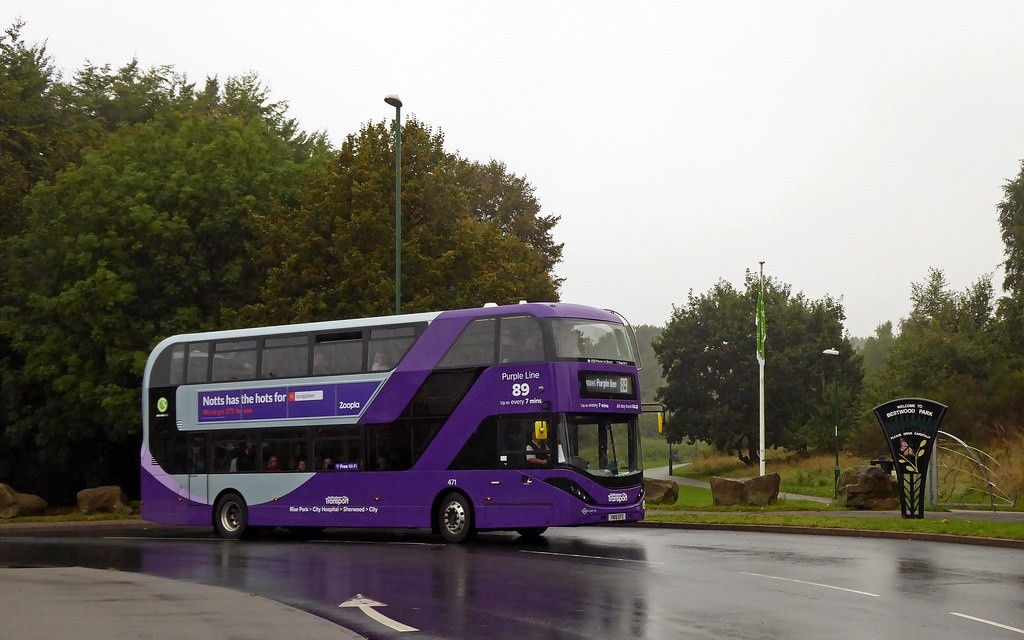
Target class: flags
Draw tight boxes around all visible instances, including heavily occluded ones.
[753,274,767,366]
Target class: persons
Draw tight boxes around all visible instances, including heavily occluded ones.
[214,441,391,472]
[525,430,551,466]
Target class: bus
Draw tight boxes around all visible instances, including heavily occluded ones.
[139,299,668,547]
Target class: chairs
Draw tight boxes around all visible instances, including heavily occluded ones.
[266,351,289,378]
[331,352,349,374]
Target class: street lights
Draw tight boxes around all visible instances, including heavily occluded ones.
[757,259,767,476]
[383,93,403,317]
[822,347,842,499]
